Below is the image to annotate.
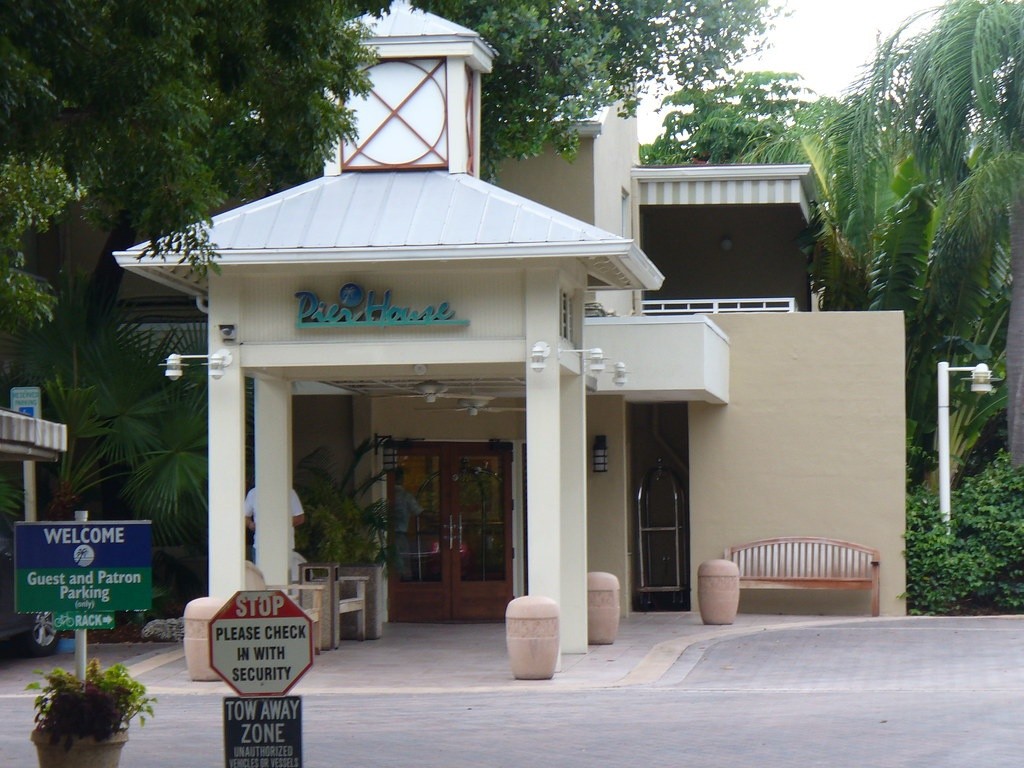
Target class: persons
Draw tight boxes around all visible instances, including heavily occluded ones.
[392,466,434,582]
[245,485,304,564]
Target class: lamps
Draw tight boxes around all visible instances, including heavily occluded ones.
[607,362,630,386]
[210,349,232,379]
[530,341,550,373]
[558,348,608,374]
[158,354,208,380]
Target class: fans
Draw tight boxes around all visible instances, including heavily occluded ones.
[415,398,525,416]
[364,381,495,403]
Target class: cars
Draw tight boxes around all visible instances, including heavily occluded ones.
[0,539,62,658]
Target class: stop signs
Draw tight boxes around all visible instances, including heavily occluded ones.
[207,589,315,698]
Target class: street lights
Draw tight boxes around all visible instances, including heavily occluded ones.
[937,361,1001,535]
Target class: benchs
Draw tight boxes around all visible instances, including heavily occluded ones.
[724,536,880,617]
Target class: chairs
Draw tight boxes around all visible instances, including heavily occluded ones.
[291,551,370,649]
[245,560,325,655]
